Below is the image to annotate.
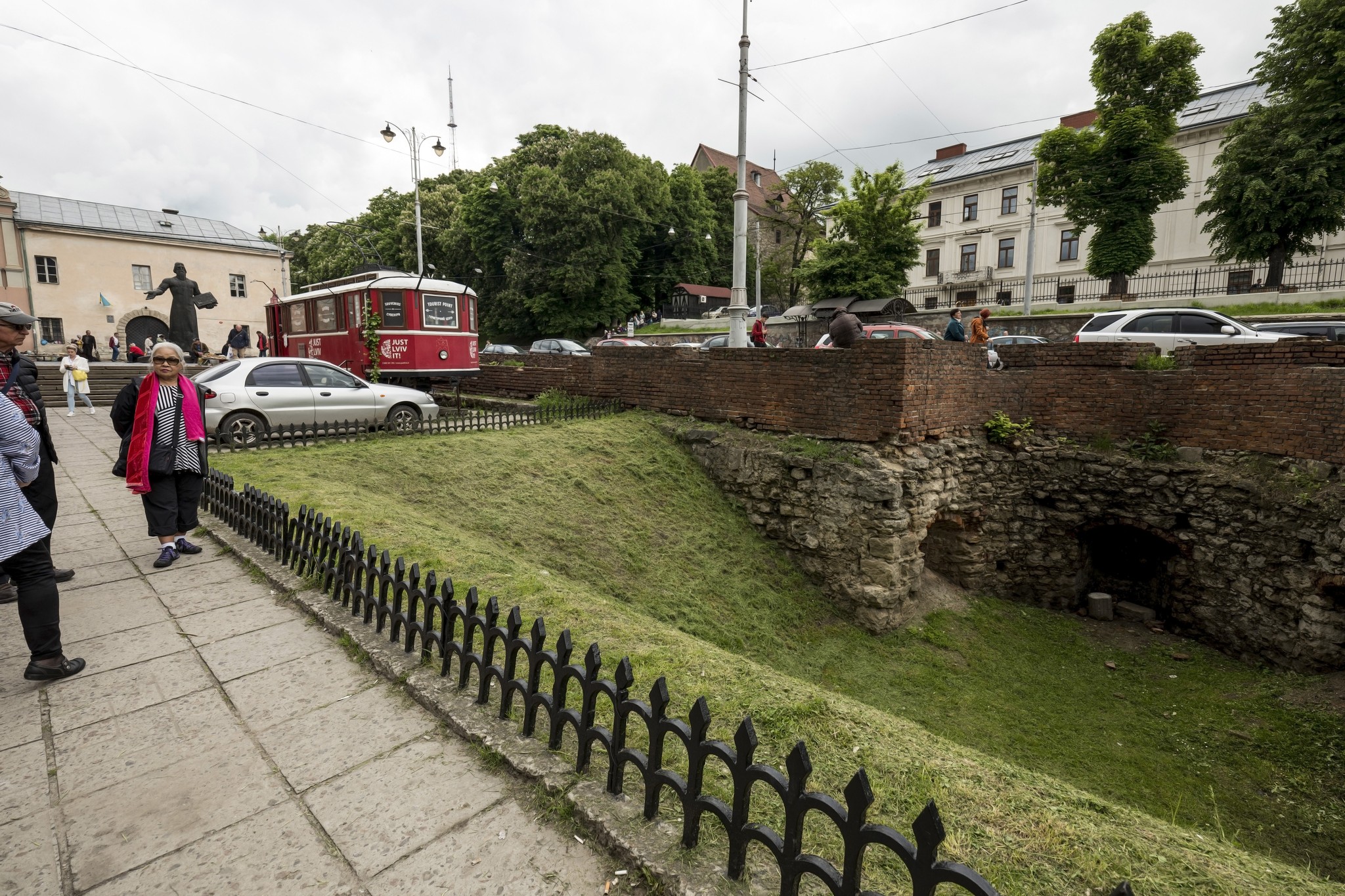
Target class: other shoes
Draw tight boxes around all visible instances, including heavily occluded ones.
[111,358,118,362]
[23,655,86,681]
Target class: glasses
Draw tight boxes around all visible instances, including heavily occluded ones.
[0,323,32,331]
[152,356,181,366]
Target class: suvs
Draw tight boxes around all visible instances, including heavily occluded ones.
[861,321,945,340]
[1072,306,1309,361]
[596,339,649,346]
[529,338,594,356]
[1250,321,1345,343]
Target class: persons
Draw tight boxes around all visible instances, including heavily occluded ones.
[71,334,84,358]
[617,318,621,327]
[625,309,662,331]
[1,390,87,682]
[109,333,120,362]
[752,313,769,348]
[82,330,96,362]
[776,342,783,348]
[59,344,96,417]
[936,332,941,336]
[143,262,218,352]
[969,308,993,346]
[0,300,77,603]
[1003,331,1010,336]
[1251,279,1262,288]
[156,335,166,343]
[652,341,659,346]
[256,331,268,357]
[109,342,208,568]
[964,335,970,343]
[222,340,229,356]
[604,324,624,339]
[944,308,965,342]
[125,343,144,363]
[829,307,867,349]
[145,335,153,354]
[485,340,492,348]
[228,324,249,359]
[191,337,210,363]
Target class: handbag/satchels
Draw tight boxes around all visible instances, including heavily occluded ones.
[73,370,88,381]
[95,349,101,362]
[79,348,83,353]
[750,335,755,342]
[226,346,233,360]
[198,357,208,365]
[148,444,176,476]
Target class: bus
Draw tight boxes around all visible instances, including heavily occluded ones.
[249,221,481,392]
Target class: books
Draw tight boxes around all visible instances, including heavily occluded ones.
[192,291,218,310]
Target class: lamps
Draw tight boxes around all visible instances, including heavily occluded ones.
[705,234,712,241]
[463,268,483,296]
[490,181,498,192]
[668,227,675,236]
[298,270,336,297]
[250,280,286,305]
[415,264,436,292]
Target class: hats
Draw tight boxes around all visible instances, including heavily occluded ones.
[0,302,42,324]
[193,337,200,342]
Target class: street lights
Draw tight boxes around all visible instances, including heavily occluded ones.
[380,119,448,275]
[257,224,300,298]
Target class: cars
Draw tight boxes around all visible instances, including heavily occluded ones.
[670,343,702,348]
[696,334,777,350]
[986,336,1056,370]
[478,344,529,354]
[814,333,889,348]
[701,306,731,319]
[747,305,780,317]
[186,356,440,449]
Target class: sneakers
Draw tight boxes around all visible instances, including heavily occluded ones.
[89,406,95,415]
[175,538,203,555]
[66,411,76,417]
[53,566,75,583]
[0,582,19,604]
[153,546,180,567]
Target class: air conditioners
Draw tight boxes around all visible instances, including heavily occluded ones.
[698,295,706,304]
